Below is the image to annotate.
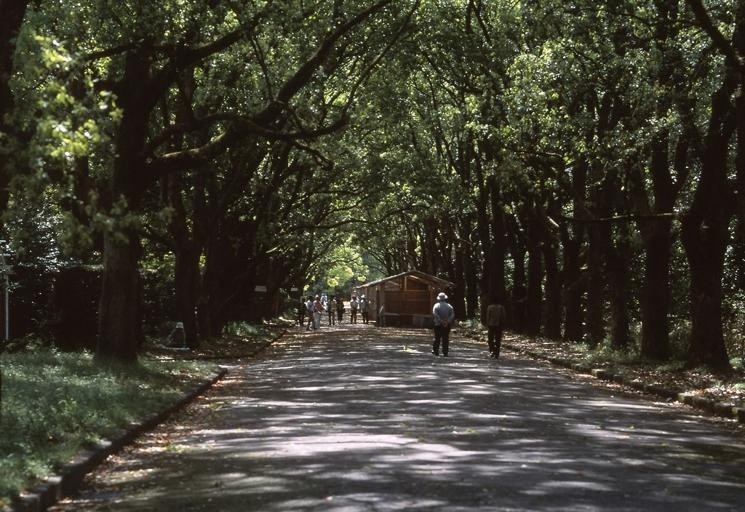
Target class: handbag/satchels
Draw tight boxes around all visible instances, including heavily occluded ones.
[339,309,345,314]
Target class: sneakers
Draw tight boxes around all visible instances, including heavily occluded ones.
[490,351,499,360]
[305,321,369,332]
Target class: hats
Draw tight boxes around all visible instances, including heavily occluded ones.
[361,294,367,298]
[436,292,448,300]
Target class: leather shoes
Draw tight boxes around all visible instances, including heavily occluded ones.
[432,350,449,357]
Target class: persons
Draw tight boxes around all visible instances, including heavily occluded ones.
[431,292,456,358]
[298,293,369,332]
[485,295,506,358]
